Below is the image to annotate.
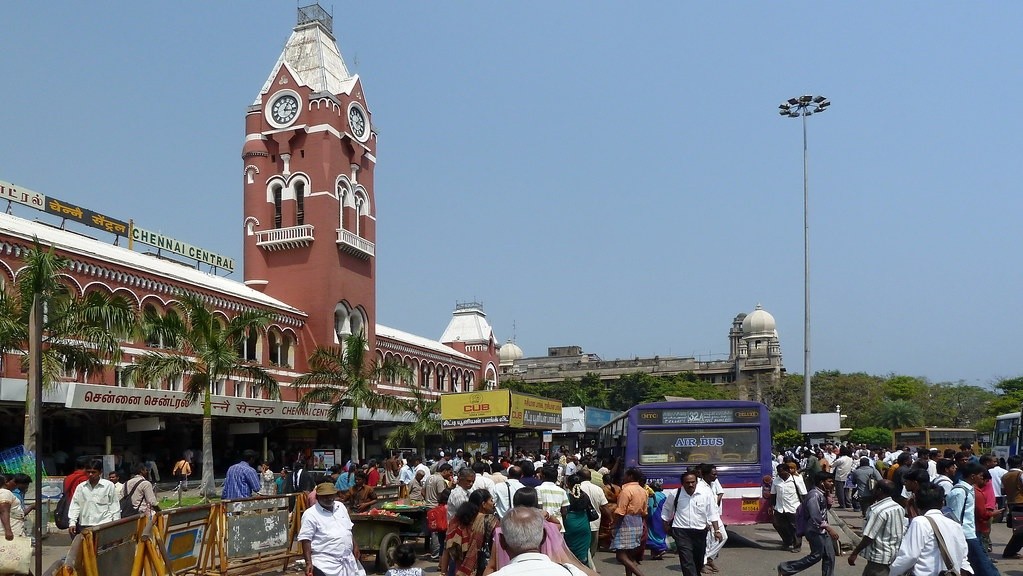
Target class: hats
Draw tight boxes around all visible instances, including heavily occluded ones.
[315,482,338,495]
[456,449,463,453]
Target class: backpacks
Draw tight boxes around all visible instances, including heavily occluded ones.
[942,485,967,525]
[795,491,821,537]
[54,492,70,529]
[120,479,146,518]
[427,503,448,530]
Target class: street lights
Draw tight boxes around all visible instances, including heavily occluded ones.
[779,94,831,414]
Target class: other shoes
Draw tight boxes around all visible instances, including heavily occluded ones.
[1003,553,1022,559]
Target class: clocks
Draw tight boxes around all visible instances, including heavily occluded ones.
[264,88,302,128]
[347,101,370,143]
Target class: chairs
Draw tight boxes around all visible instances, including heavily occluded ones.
[688,453,742,462]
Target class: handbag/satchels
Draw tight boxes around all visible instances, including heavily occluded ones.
[476,545,491,576]
[176,468,182,477]
[868,478,877,490]
[587,505,599,522]
[1006,511,1023,528]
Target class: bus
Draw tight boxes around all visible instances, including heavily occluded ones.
[990,411,1023,468]
[891,426,979,463]
[596,399,777,525]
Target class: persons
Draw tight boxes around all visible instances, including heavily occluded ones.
[330,449,431,513]
[259,461,274,511]
[384,544,426,576]
[297,483,367,576]
[63,455,92,541]
[183,445,194,480]
[0,473,36,576]
[221,450,260,562]
[119,445,160,483]
[275,469,289,509]
[68,459,121,535]
[109,471,122,488]
[195,446,203,480]
[768,440,1023,576]
[268,442,343,491]
[120,462,161,518]
[173,456,192,491]
[420,445,603,576]
[598,455,728,576]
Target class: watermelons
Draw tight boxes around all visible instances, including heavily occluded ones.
[383,503,396,509]
